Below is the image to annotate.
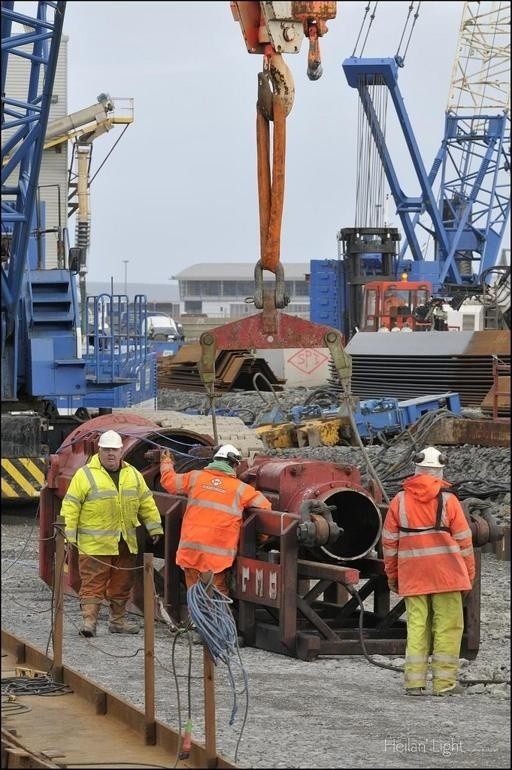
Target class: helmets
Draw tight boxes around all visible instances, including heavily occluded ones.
[415,448,447,469]
[212,444,240,460]
[97,430,123,449]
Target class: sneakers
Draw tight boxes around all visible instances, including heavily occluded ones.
[406,687,423,697]
[432,685,465,696]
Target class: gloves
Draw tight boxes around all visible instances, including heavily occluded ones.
[388,577,399,595]
[159,448,171,462]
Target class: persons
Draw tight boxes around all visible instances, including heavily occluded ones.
[157,444,273,651]
[382,446,476,697]
[60,428,165,638]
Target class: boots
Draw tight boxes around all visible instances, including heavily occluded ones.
[108,599,140,635]
[77,599,102,638]
